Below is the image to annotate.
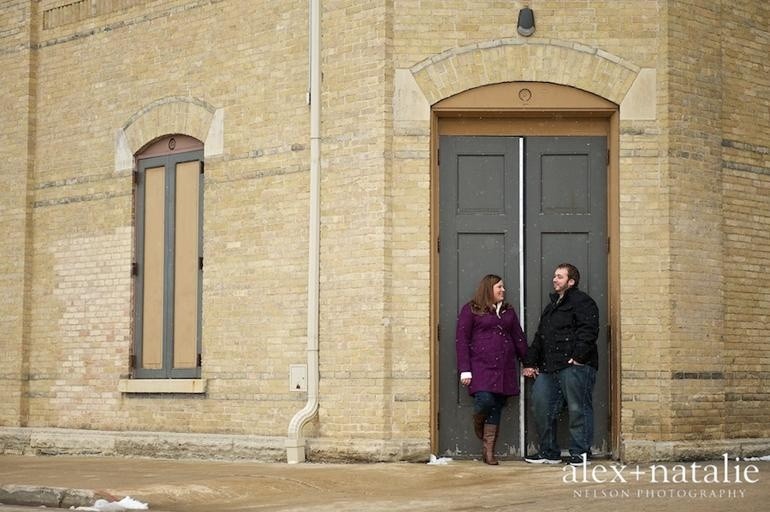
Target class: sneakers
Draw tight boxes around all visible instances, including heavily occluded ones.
[569,453,592,466]
[524,453,563,464]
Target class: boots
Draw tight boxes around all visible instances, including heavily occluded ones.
[474,414,484,440]
[482,423,499,465]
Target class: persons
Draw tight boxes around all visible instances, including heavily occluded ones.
[456,275,539,464]
[522,264,599,464]
